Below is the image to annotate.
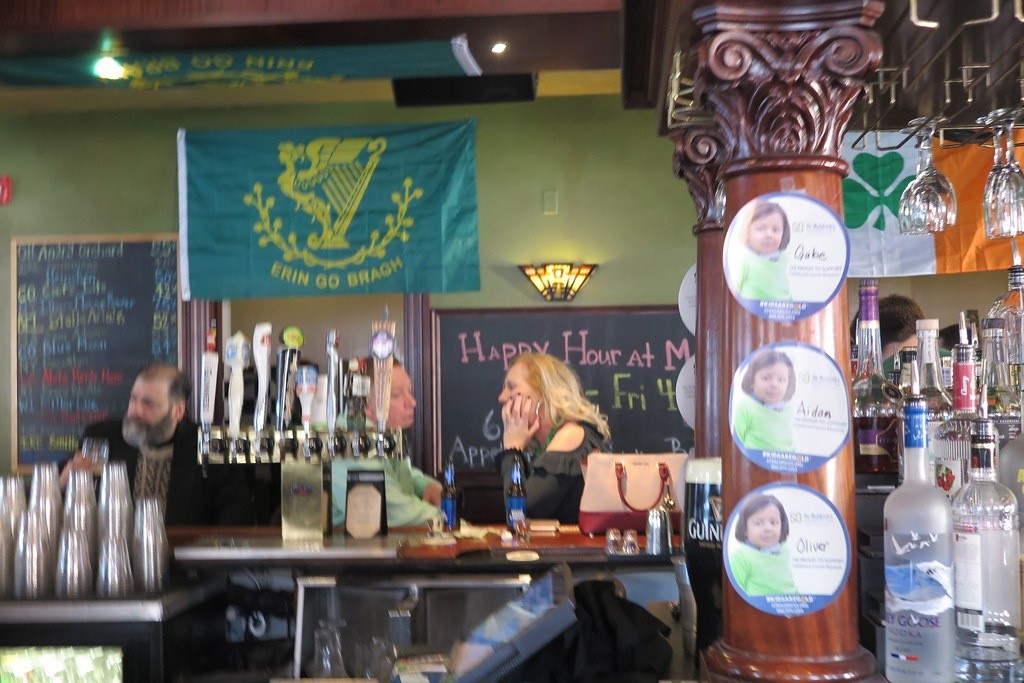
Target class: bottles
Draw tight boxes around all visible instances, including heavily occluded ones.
[506,456,527,536]
[441,461,456,534]
[851,265,1023,682]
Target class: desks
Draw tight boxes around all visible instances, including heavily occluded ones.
[170,525,683,567]
[0,580,220,683]
[292,571,1024,683]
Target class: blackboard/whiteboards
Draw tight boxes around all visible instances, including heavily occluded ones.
[431,305,696,487]
[9,235,180,474]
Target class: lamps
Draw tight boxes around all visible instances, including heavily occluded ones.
[518,264,600,300]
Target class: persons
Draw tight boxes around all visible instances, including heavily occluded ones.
[938,322,974,352]
[848,293,922,360]
[735,494,797,595]
[737,201,795,301]
[735,349,803,452]
[330,352,450,526]
[54,363,201,525]
[497,350,614,522]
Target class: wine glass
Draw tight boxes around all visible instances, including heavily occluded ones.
[898,116,956,235]
[976,108,1024,240]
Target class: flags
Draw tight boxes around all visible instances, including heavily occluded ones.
[838,128,1023,277]
[172,128,484,295]
[0,35,484,95]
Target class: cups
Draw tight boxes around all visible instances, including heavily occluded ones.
[670,556,697,656]
[622,530,639,556]
[605,529,623,556]
[684,456,724,670]
[0,438,168,600]
